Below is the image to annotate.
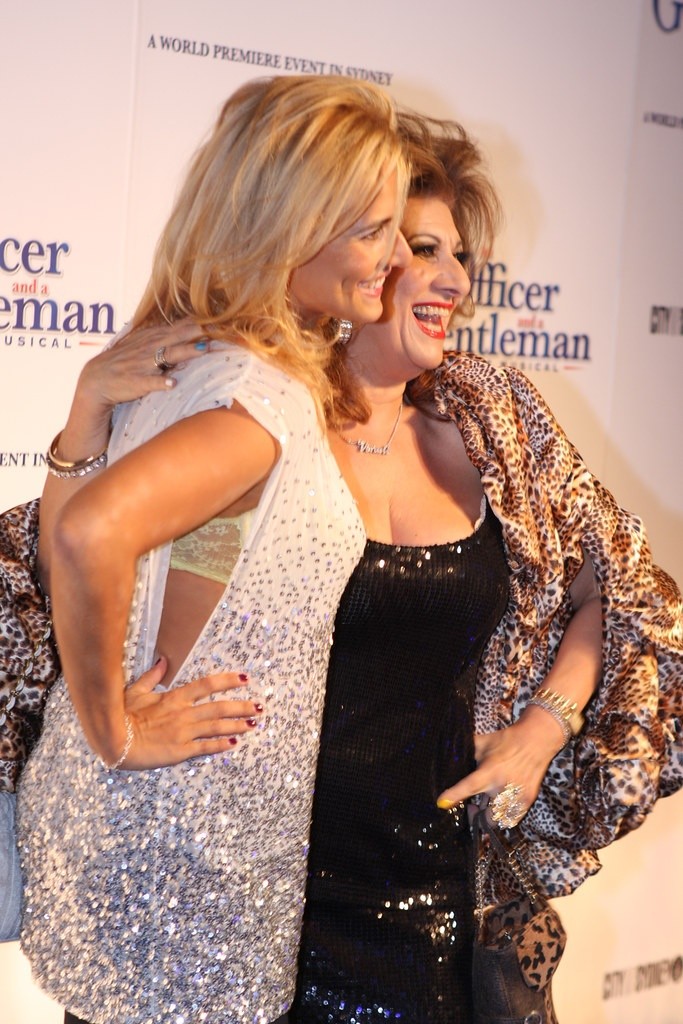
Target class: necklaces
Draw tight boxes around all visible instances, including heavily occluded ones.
[331,403,403,455]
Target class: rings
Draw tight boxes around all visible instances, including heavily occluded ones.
[153,347,174,369]
[487,781,528,829]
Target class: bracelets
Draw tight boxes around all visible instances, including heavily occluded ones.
[100,712,134,772]
[46,430,108,478]
[524,688,582,748]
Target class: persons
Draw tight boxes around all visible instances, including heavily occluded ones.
[35,114,683,1024]
[17,77,416,1024]
